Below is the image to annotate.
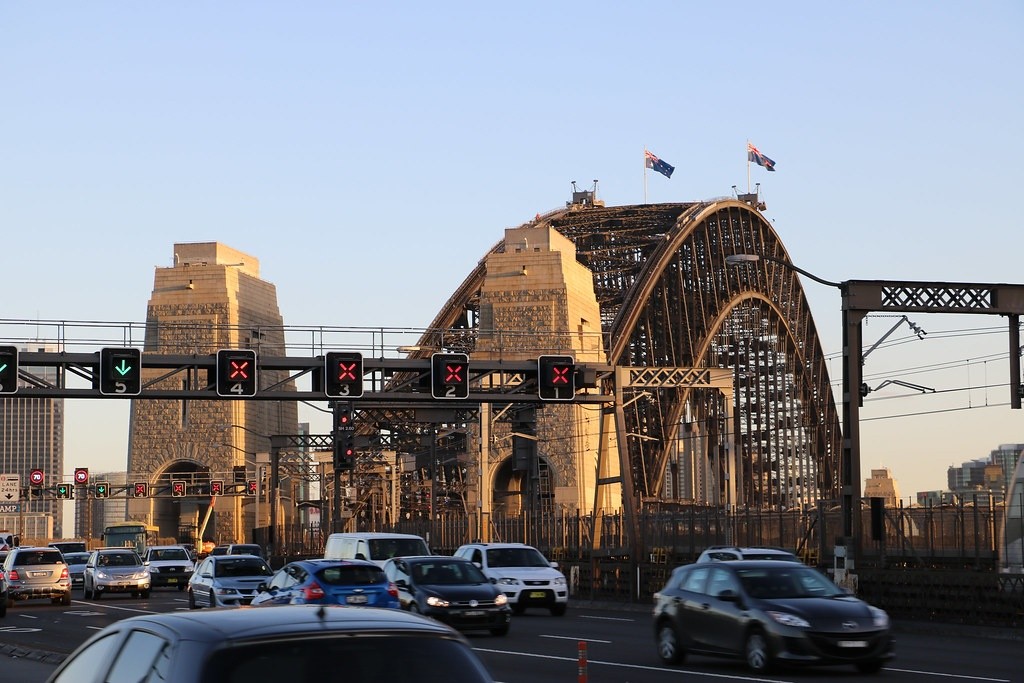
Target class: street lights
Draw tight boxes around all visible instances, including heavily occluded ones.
[724,253,851,535]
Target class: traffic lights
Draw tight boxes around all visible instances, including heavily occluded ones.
[341,431,355,459]
[338,406,351,427]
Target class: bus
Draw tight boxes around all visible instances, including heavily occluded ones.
[100,522,160,555]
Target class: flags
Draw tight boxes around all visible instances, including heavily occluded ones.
[645,151,675,179]
[749,145,776,171]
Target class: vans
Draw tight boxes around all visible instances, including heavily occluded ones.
[207,544,263,569]
[323,532,450,582]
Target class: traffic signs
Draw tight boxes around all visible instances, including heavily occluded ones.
[0,474,20,502]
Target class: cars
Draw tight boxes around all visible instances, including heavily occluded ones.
[380,556,513,638]
[0,532,200,618]
[650,559,892,677]
[43,603,500,683]
[187,555,276,611]
[249,556,402,610]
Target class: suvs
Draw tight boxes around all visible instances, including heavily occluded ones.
[451,542,570,617]
[688,544,822,592]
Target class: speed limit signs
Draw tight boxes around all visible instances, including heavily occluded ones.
[75,470,88,483]
[31,470,44,483]
